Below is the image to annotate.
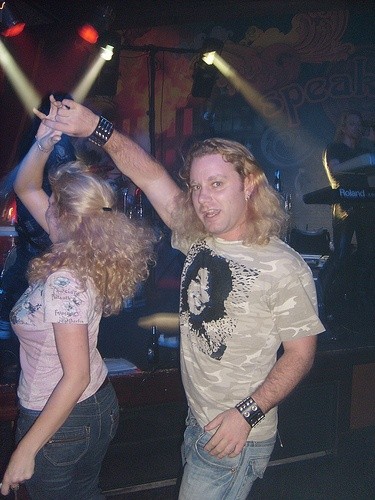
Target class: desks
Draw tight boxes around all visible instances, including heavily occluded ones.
[1,326,375,500]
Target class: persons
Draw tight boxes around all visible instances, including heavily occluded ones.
[0,95,120,500]
[328,109,374,307]
[41,98,326,500]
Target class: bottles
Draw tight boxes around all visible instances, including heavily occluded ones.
[282,191,289,211]
[288,192,292,211]
[273,168,281,192]
[144,326,160,372]
[128,188,143,222]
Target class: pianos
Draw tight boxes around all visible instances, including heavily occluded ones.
[304,184,375,204]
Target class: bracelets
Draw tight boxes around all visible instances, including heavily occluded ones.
[237,396,266,430]
[36,136,56,154]
[86,116,117,147]
